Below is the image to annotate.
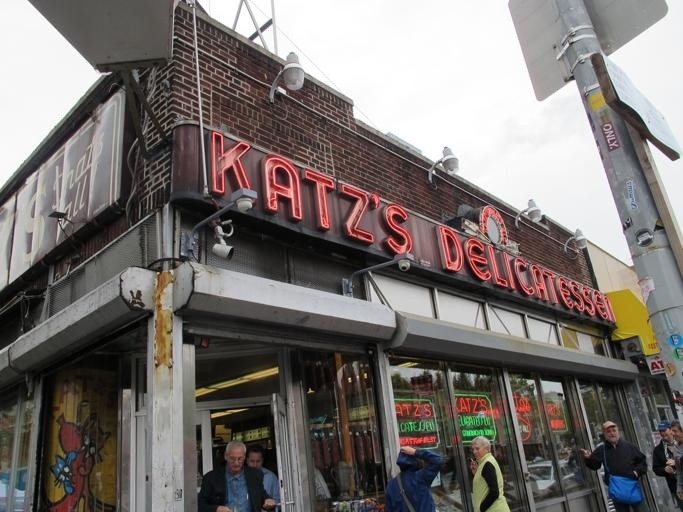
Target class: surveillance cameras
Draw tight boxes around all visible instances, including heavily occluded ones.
[236,197,253,214]
[211,225,235,260]
[398,259,410,272]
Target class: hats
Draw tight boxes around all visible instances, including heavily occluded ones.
[603,421,616,429]
[657,422,671,430]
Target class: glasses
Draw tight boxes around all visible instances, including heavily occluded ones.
[229,457,244,462]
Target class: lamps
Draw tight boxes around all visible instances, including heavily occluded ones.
[342,250,415,293]
[563,227,587,261]
[186,185,258,251]
[513,198,542,230]
[267,51,307,122]
[424,145,460,192]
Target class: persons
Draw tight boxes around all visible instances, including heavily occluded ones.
[470,435,510,512]
[580,420,647,512]
[198,441,280,512]
[384,446,443,512]
[652,419,683,512]
[314,468,332,512]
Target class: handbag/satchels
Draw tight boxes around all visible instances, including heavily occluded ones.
[608,476,644,504]
